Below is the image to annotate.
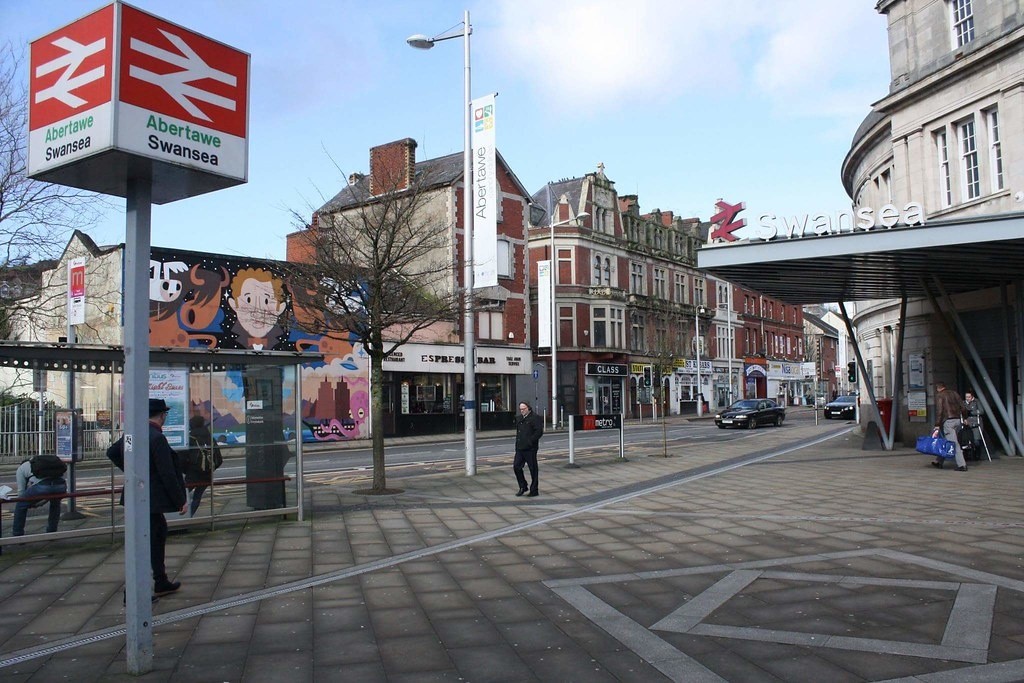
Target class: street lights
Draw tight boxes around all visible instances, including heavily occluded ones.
[695,303,706,418]
[404,8,477,479]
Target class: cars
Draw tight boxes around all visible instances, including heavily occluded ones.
[714,398,785,430]
[823,396,858,421]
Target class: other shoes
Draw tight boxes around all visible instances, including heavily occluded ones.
[527,490,538,497]
[954,464,967,471]
[515,486,528,496]
[931,461,942,468]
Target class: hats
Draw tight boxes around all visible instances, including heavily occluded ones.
[190,416,204,429]
[519,400,532,408]
[148,398,171,416]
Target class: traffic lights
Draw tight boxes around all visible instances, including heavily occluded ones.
[848,360,858,383]
[643,366,653,388]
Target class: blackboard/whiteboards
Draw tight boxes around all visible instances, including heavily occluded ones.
[416,385,436,402]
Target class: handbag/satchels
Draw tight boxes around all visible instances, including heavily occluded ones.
[201,444,223,471]
[958,425,975,450]
[916,429,956,459]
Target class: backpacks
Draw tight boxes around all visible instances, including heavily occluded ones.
[21,455,68,485]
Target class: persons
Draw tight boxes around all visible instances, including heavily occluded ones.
[701,393,705,405]
[12,459,66,536]
[106,398,187,605]
[806,388,813,403]
[513,401,543,496]
[185,415,222,516]
[931,382,968,471]
[963,391,983,459]
[458,395,465,416]
[789,387,794,404]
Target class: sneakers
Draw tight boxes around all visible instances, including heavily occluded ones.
[123,590,157,606]
[154,579,181,598]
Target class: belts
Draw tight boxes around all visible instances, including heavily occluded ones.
[968,414,983,417]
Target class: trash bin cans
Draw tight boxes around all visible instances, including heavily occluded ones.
[876,397,898,442]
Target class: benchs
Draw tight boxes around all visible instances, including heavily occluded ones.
[0,475,290,537]
